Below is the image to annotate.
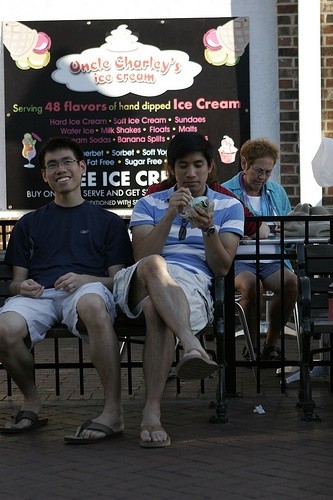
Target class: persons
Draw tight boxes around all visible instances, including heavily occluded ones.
[219,137,298,361]
[145,140,270,380]
[115,131,244,447]
[0,137,135,444]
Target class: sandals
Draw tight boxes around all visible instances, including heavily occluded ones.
[263,338,282,361]
[241,346,257,361]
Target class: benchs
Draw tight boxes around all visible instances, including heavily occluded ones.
[0,264,228,424]
[297,242,333,422]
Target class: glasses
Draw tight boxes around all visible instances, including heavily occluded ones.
[46,160,77,170]
[178,219,189,241]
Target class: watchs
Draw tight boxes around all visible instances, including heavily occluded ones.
[202,224,217,237]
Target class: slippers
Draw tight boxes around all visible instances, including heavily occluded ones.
[139,425,171,448]
[64,419,122,443]
[0,411,48,433]
[176,348,218,382]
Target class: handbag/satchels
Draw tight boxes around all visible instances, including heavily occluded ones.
[284,204,330,238]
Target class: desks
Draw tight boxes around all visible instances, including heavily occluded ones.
[240,236,330,386]
